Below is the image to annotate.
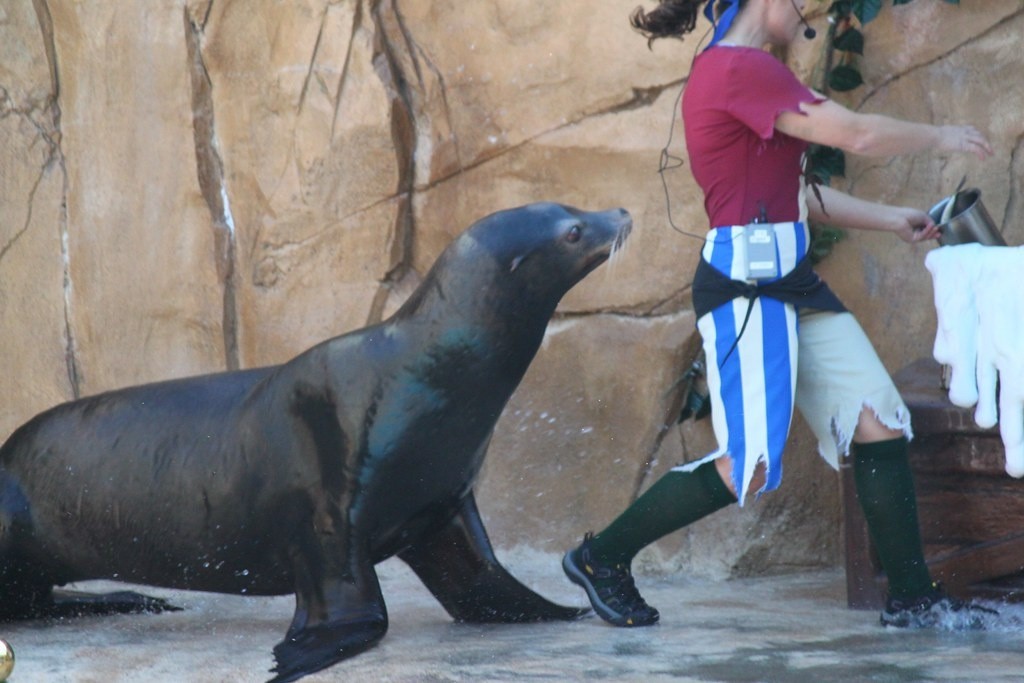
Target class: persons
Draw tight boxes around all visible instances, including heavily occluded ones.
[561,0,995,630]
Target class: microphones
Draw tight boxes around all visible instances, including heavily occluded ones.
[792,0,816,39]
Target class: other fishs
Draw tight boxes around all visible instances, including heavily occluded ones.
[940,174,968,224]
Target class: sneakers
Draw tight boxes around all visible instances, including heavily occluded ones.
[880,587,1002,631]
[562,532,662,626]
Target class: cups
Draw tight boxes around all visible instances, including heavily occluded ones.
[927,188,1008,246]
